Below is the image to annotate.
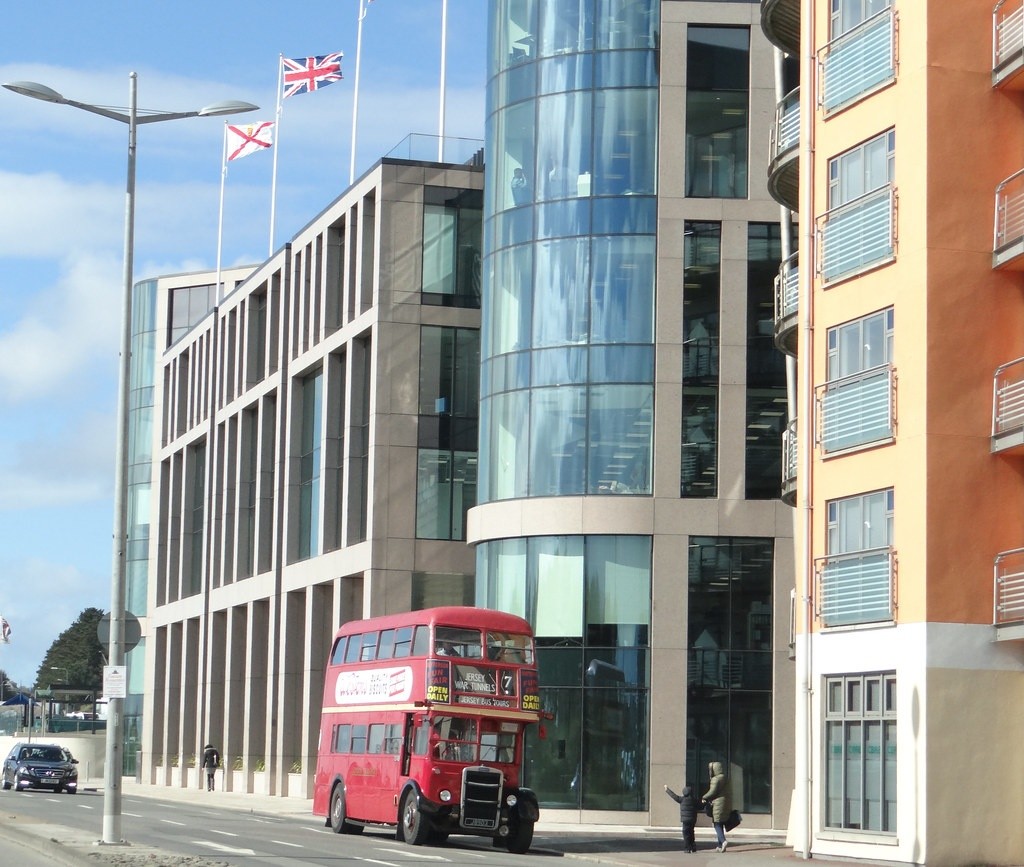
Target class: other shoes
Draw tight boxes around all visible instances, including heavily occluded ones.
[716,847,721,853]
[721,840,728,853]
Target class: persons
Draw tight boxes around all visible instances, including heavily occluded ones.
[702,761,732,853]
[664,785,705,853]
[436,635,461,657]
[202,745,220,792]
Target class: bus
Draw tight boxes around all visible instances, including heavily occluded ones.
[580,659,629,797]
[312,607,548,854]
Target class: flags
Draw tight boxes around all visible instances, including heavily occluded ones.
[284,52,344,99]
[227,121,276,161]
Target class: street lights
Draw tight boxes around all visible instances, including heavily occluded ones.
[0,73,260,848]
[51,667,68,685]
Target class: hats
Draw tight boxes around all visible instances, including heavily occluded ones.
[205,744,213,748]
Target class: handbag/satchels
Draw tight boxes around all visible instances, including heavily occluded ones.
[724,810,742,832]
[213,752,221,767]
[706,801,713,818]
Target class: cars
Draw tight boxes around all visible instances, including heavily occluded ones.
[0,742,79,794]
